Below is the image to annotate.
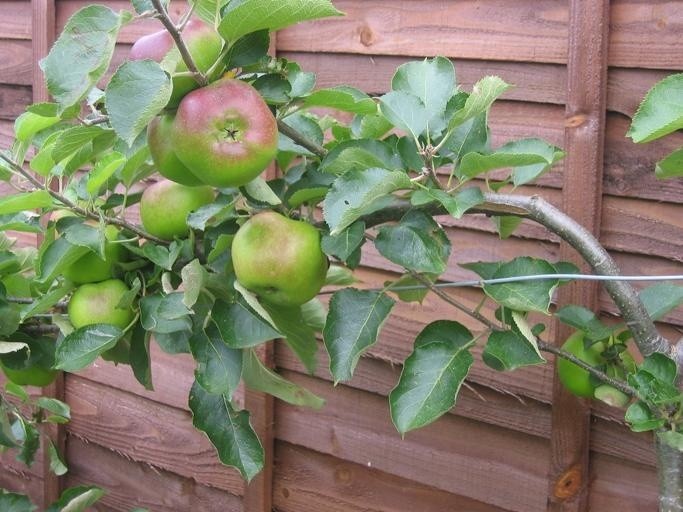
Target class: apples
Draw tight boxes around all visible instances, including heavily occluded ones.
[66,279,136,335]
[38,198,122,282]
[0,358,56,385]
[231,210,328,307]
[141,179,215,241]
[557,327,640,400]
[130,17,280,188]
